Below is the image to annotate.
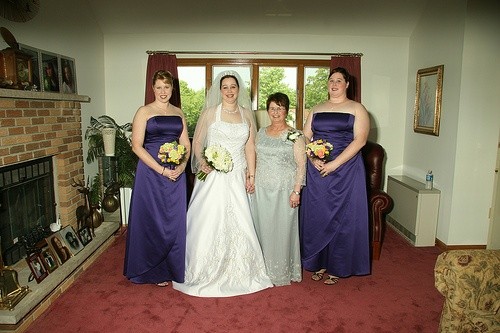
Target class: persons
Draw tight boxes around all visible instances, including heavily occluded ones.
[173,70,273,297]
[123,70,191,287]
[298,67,371,286]
[247,92,307,286]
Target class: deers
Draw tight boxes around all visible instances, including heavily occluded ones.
[70,175,96,241]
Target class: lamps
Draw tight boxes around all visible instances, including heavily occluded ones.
[102,128,117,181]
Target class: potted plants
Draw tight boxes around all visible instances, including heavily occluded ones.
[85,115,139,212]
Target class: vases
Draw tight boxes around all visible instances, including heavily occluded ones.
[87,207,102,228]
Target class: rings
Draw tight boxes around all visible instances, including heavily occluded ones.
[208,166,211,168]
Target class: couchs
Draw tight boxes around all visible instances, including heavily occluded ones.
[434,249,500,333]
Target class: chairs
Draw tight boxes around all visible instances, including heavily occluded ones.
[361,142,392,261]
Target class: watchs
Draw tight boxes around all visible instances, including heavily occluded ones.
[292,190,300,195]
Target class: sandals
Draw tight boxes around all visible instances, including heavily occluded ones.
[324,275,339,285]
[312,271,326,280]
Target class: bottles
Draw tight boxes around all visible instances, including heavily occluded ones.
[425,171,433,190]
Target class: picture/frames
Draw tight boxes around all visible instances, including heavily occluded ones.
[413,64,443,136]
[45,232,73,266]
[77,226,92,246]
[60,224,85,256]
[25,253,48,284]
[39,248,59,273]
[16,43,77,94]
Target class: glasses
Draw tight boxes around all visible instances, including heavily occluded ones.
[270,107,286,112]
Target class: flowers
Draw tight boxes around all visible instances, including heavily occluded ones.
[288,128,303,143]
[158,140,187,165]
[306,139,333,161]
[195,145,236,181]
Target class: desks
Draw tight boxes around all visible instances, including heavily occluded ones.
[385,175,441,247]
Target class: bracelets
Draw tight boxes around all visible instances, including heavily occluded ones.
[250,175,255,177]
[161,167,166,175]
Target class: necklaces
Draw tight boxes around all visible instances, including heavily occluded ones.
[222,107,239,114]
